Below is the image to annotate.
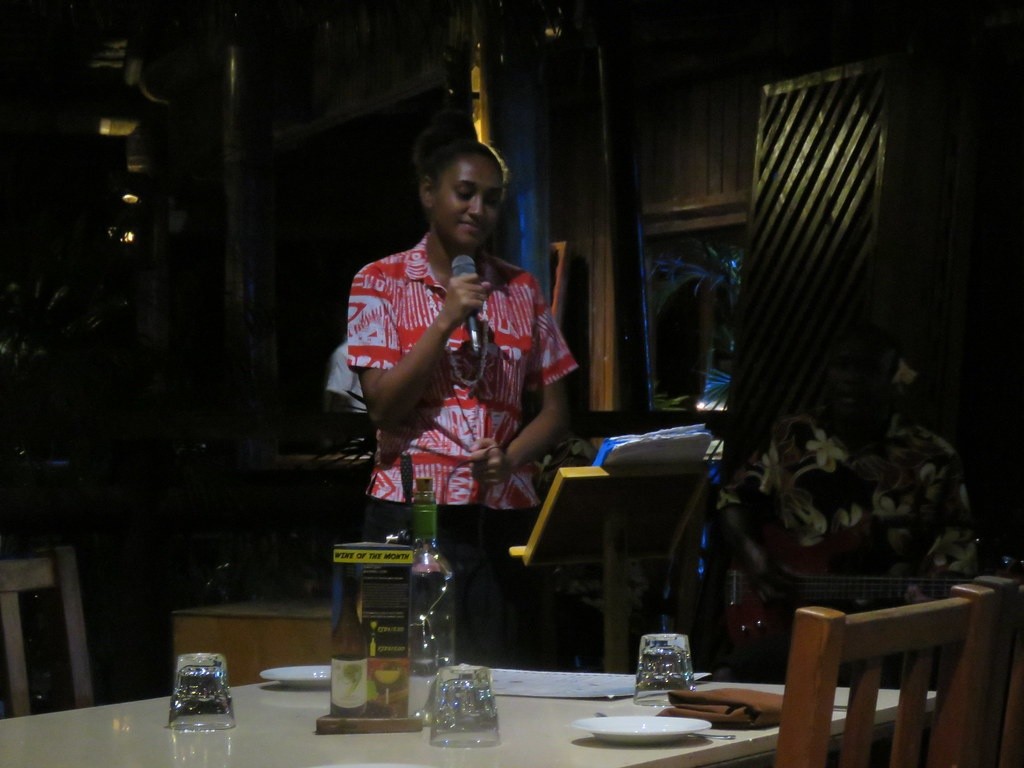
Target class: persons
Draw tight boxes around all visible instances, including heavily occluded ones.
[714,326,979,690]
[320,108,581,672]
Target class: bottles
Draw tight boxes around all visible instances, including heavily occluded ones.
[409,480,454,676]
[331,563,368,718]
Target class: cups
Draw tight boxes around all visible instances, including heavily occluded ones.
[634,633,696,706]
[430,666,499,746]
[169,653,235,731]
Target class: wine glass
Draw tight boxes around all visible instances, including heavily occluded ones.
[374,662,399,705]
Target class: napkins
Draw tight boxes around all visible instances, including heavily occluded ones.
[658,685,784,728]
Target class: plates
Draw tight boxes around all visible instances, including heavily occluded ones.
[567,717,711,744]
[260,666,331,687]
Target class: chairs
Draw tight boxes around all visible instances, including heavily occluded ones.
[776,583,995,768]
[974,576,1024,768]
[0,546,94,719]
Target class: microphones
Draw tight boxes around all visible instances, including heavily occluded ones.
[452,255,482,357]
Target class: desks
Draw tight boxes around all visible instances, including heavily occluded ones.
[172,607,334,686]
[0,681,937,768]
[273,452,374,586]
